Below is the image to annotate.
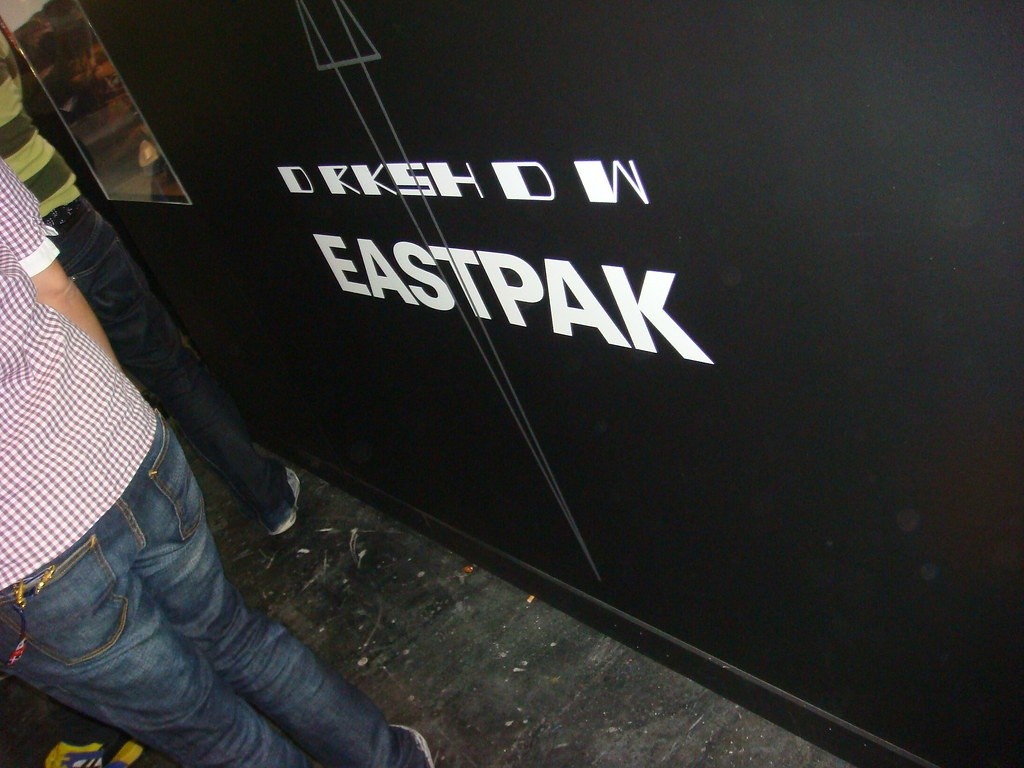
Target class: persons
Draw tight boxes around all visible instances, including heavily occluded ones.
[0,156,435,768]
[0,22,301,536]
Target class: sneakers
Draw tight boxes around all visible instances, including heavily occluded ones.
[268,465,301,534]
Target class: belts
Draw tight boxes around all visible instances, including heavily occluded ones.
[43,194,88,226]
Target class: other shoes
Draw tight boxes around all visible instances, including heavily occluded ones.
[388,724,435,768]
